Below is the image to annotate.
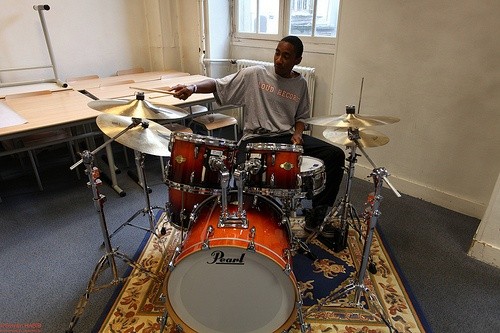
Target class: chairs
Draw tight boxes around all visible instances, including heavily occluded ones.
[162,123,193,133]
[21,130,81,192]
[116,68,145,76]
[193,104,238,138]
[65,75,100,82]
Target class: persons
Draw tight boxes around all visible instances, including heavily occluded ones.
[167,37,345,238]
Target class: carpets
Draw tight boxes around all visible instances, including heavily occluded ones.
[89,207,431,333]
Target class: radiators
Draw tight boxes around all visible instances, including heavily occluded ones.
[239,59,316,137]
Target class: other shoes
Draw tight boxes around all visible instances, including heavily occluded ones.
[305,203,341,237]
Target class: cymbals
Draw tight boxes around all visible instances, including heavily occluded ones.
[87,92,188,120]
[299,104,401,127]
[322,128,391,148]
[95,112,174,157]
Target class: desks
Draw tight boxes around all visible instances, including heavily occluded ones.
[0,69,217,137]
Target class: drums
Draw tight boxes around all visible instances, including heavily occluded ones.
[165,130,238,195]
[245,141,304,195]
[160,190,301,333]
[164,185,229,233]
[300,154,327,196]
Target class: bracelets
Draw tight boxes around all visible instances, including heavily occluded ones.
[191,83,197,93]
